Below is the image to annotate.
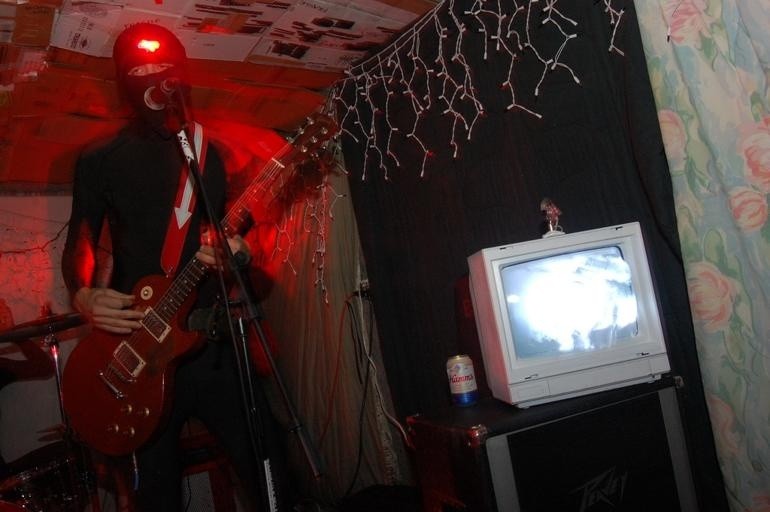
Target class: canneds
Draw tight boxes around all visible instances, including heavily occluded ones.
[445,352,478,409]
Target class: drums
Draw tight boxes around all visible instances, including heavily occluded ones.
[0,458,94,512]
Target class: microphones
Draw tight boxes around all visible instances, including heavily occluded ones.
[144,78,176,111]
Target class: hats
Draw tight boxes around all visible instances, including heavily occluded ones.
[113,22,187,74]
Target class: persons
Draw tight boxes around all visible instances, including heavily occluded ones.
[58,21,256,512]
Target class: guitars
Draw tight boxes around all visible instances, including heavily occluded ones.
[60,114,337,458]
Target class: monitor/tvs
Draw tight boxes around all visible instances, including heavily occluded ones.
[468,222,672,409]
